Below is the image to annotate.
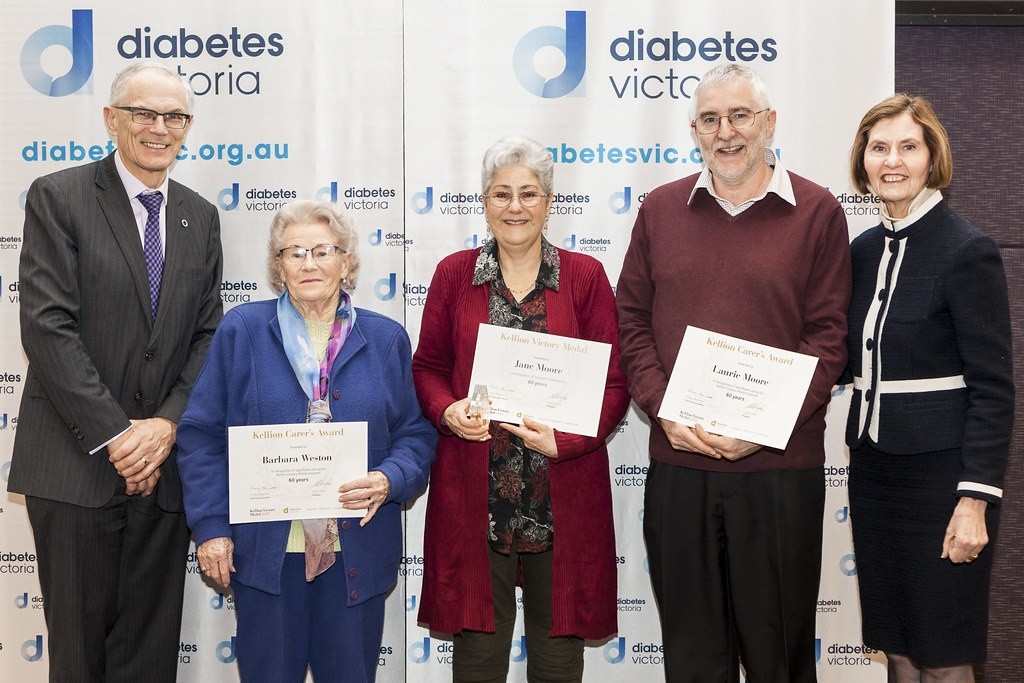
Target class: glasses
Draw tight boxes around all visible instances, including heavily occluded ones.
[278,244,347,264]
[487,191,549,208]
[695,107,770,134]
[115,107,190,129]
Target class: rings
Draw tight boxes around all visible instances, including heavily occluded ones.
[461,431,464,438]
[201,566,211,571]
[369,498,375,509]
[968,554,979,561]
[141,458,148,466]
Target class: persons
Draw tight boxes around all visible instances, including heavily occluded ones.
[834,94,1015,683]
[174,200,437,683]
[615,63,852,683]
[410,137,631,683]
[6,63,223,683]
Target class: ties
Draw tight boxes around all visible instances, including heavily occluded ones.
[135,191,164,327]
